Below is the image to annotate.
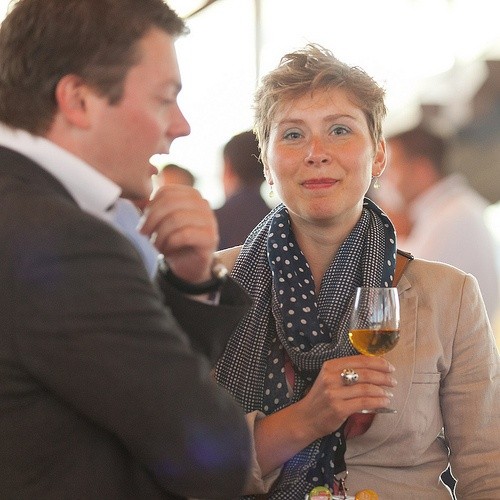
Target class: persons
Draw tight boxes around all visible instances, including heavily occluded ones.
[212,44,500,500]
[0,0,253,500]
[382,130,500,325]
[213,131,272,251]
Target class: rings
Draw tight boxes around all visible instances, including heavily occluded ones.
[341,367,360,384]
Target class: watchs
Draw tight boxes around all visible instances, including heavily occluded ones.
[160,257,229,294]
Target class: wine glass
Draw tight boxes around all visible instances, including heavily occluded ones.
[348,287,401,414]
